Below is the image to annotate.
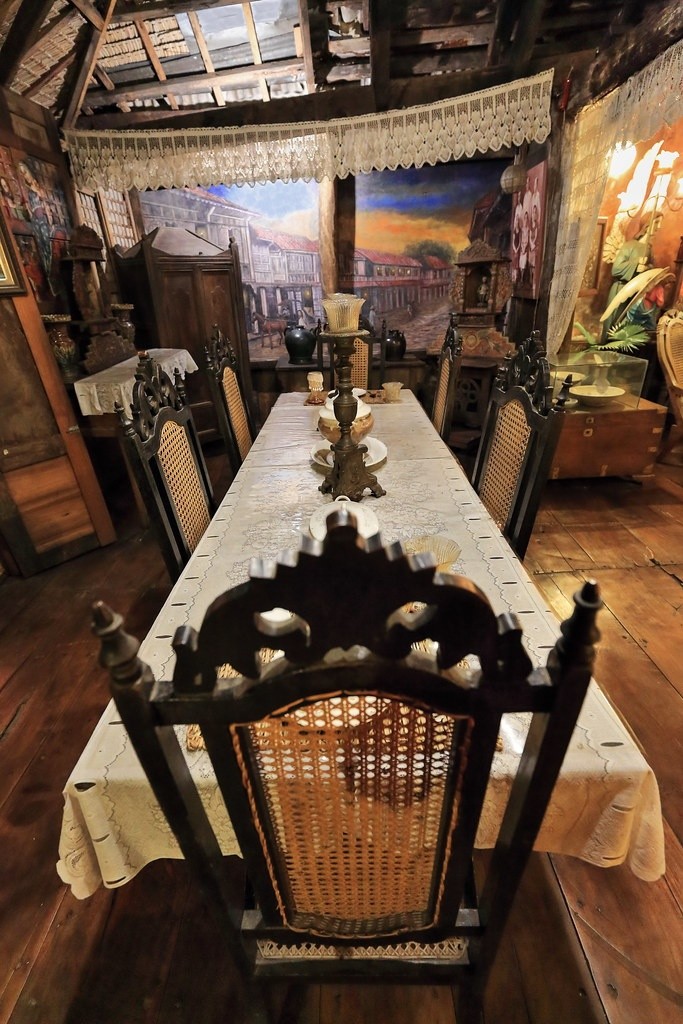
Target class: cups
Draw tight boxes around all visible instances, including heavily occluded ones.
[381,382,404,400]
[404,533,463,571]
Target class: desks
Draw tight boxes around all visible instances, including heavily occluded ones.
[52,378,668,911]
[275,352,427,393]
[72,349,198,528]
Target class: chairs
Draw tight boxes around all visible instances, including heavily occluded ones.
[92,496,606,1024]
[114,351,219,584]
[203,318,256,474]
[470,328,576,559]
[315,334,388,391]
[431,326,463,441]
[655,310,683,463]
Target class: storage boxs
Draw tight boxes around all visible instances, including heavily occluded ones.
[546,394,668,481]
[544,350,649,411]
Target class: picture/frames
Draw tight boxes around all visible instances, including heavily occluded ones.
[569,217,608,296]
[0,208,30,298]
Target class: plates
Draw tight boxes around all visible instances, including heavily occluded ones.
[309,437,388,469]
[328,388,368,399]
[270,649,393,732]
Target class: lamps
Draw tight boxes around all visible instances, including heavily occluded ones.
[318,292,388,501]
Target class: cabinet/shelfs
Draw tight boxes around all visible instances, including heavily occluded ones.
[113,227,257,445]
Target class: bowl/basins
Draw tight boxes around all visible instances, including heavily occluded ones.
[569,385,625,407]
[551,398,578,408]
